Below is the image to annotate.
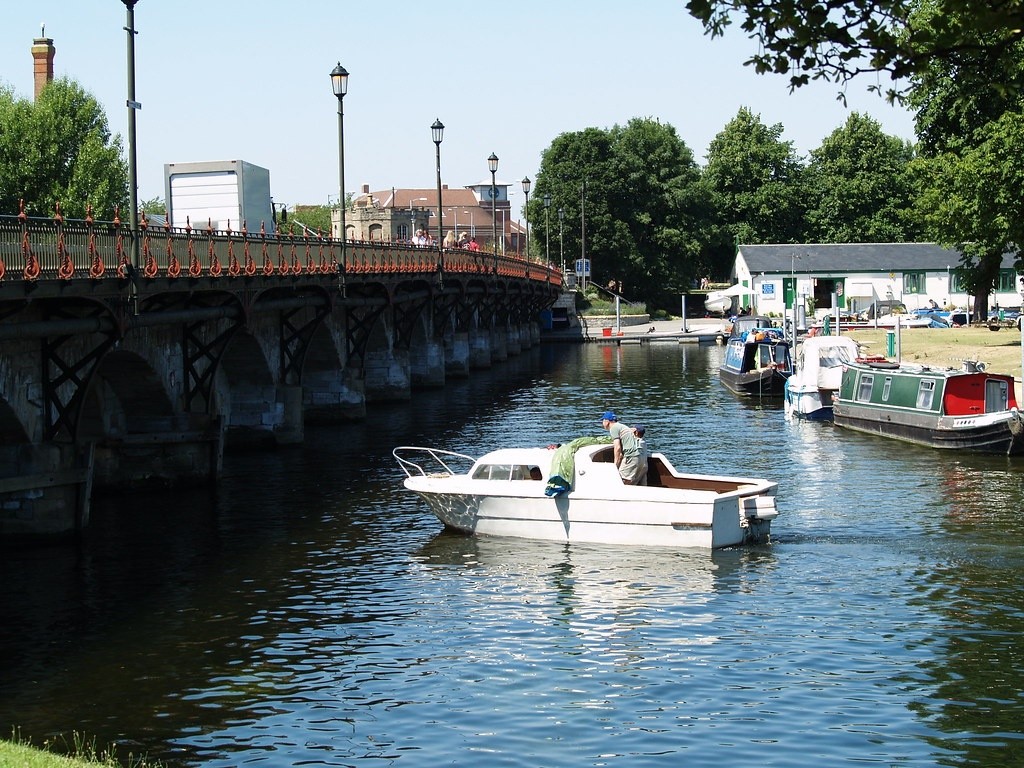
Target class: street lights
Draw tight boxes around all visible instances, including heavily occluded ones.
[522,175,531,284]
[410,198,427,238]
[448,208,458,243]
[487,151,500,283]
[431,117,445,290]
[495,208,505,255]
[558,207,566,284]
[464,211,473,238]
[542,194,552,291]
[329,60,350,298]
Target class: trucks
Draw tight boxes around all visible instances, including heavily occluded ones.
[164,159,287,237]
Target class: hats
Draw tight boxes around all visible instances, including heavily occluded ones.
[633,424,645,436]
[600,411,617,421]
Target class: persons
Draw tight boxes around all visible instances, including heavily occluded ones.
[929,299,938,309]
[700,278,708,290]
[411,229,479,253]
[599,411,648,487]
[740,307,751,315]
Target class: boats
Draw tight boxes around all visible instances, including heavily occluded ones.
[830,355,1023,456]
[392,446,780,548]
[719,316,859,423]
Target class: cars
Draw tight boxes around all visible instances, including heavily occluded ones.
[823,311,859,323]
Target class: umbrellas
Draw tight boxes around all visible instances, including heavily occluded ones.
[718,284,758,302]
